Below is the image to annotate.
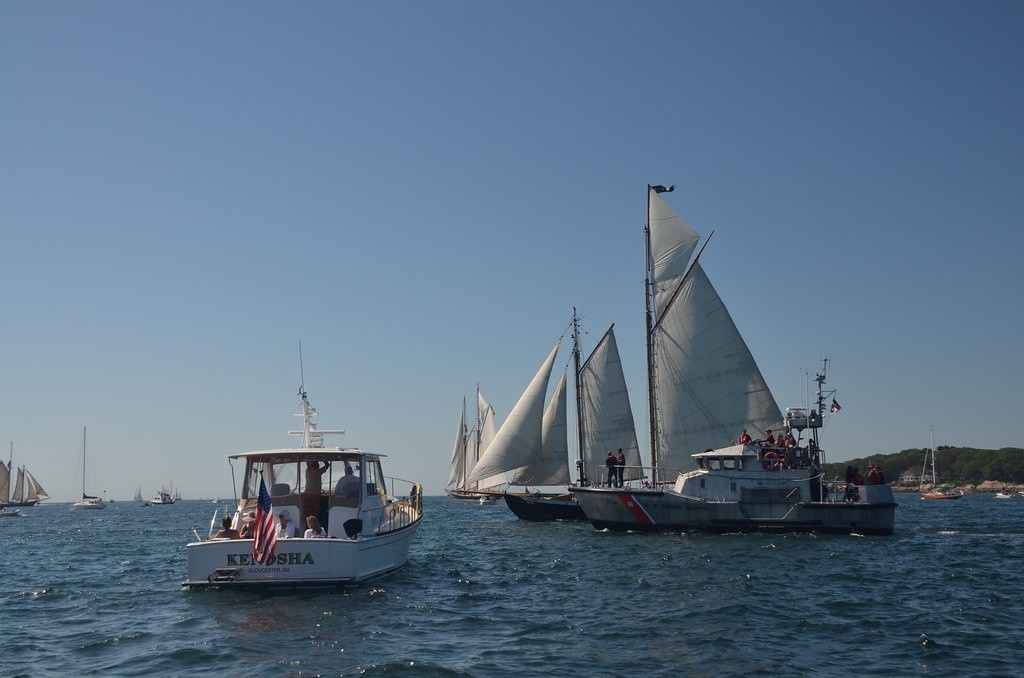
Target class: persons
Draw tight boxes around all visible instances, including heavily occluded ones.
[617,448,625,488]
[334,466,360,495]
[643,481,651,489]
[766,429,797,470]
[810,410,817,418]
[845,465,883,503]
[215,517,240,540]
[738,429,751,444]
[275,509,295,538]
[304,516,326,538]
[304,461,330,524]
[239,511,256,540]
[605,451,618,488]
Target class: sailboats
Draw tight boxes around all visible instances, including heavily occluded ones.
[0,441,52,506]
[920,424,963,500]
[440,183,900,538]
[132,479,183,504]
[72,425,106,510]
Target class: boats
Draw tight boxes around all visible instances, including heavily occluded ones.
[0,509,20,516]
[180,340,425,589]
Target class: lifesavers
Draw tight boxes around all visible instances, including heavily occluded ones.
[764,452,780,469]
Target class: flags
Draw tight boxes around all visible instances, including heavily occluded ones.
[830,398,841,415]
[253,470,277,566]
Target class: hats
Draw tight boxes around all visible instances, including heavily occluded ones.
[242,511,256,522]
[279,509,289,520]
